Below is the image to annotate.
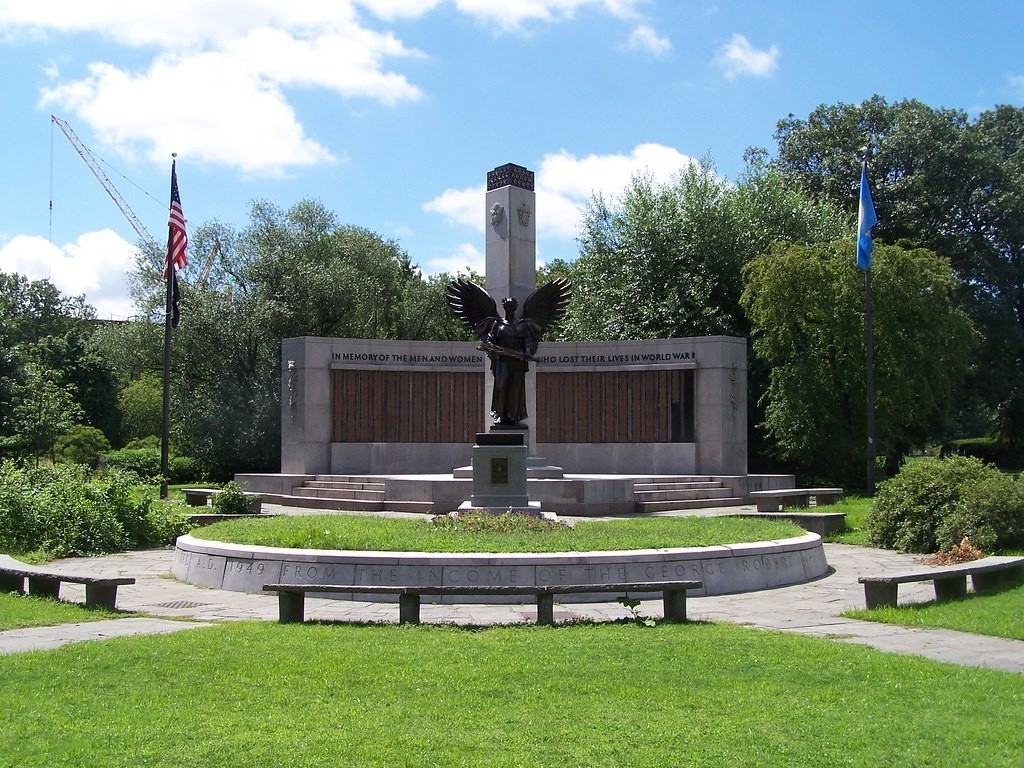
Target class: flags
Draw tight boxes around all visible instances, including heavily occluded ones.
[164,175,188,280]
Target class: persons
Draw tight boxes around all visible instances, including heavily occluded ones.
[480,296,535,424]
[856,162,879,271]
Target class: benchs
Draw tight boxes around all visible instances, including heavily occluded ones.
[800,488,843,508]
[0,552,136,611]
[750,490,808,512]
[738,512,850,538]
[965,555,1024,592]
[262,584,407,625]
[181,488,268,514]
[407,585,545,626]
[858,564,1006,610]
[546,580,703,626]
